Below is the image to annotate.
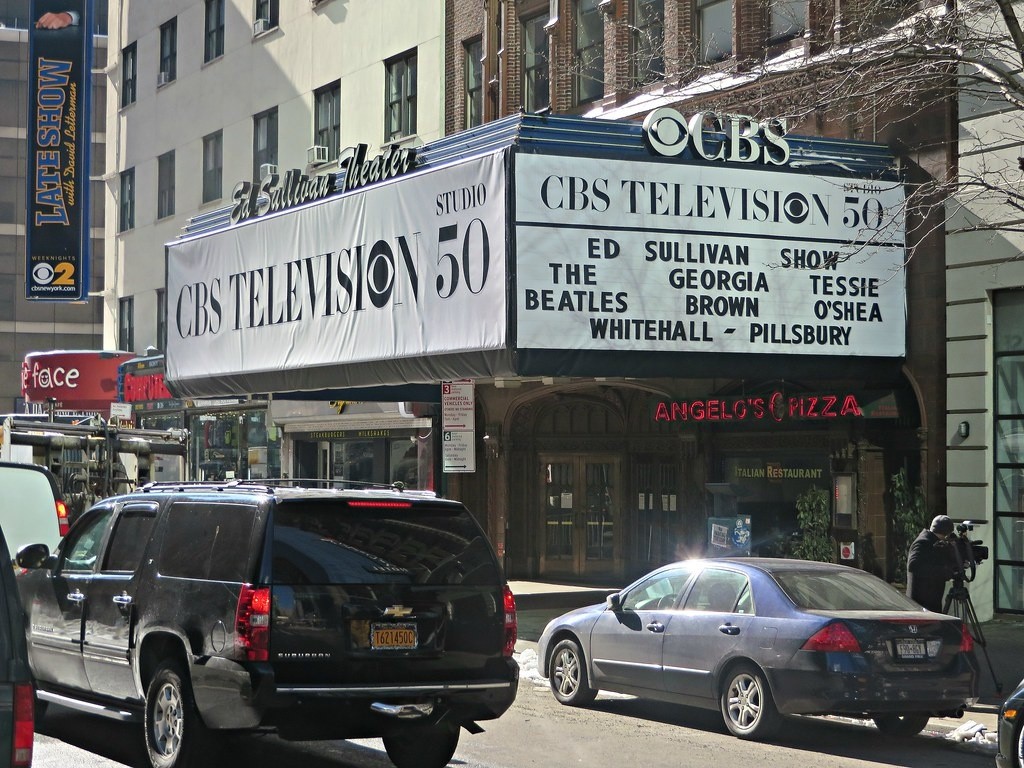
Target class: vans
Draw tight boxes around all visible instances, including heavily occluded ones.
[0,526,36,768]
[0,460,70,578]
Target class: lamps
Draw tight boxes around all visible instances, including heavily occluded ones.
[958,421,969,439]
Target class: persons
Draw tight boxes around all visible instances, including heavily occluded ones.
[906,515,969,614]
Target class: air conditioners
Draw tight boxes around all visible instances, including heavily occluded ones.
[157,72,168,88]
[252,19,269,36]
[260,163,278,181]
[307,145,328,164]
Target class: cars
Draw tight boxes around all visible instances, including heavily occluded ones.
[994,677,1024,767]
[538,555,979,741]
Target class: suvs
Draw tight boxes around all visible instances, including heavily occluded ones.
[14,479,521,768]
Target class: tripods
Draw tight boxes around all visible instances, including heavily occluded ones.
[942,572,1003,698]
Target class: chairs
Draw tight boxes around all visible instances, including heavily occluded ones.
[704,583,739,613]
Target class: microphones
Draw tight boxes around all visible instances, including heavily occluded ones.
[967,540,983,545]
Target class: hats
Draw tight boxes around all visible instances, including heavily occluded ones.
[930,515,954,535]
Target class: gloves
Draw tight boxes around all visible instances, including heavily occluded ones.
[944,565,955,581]
